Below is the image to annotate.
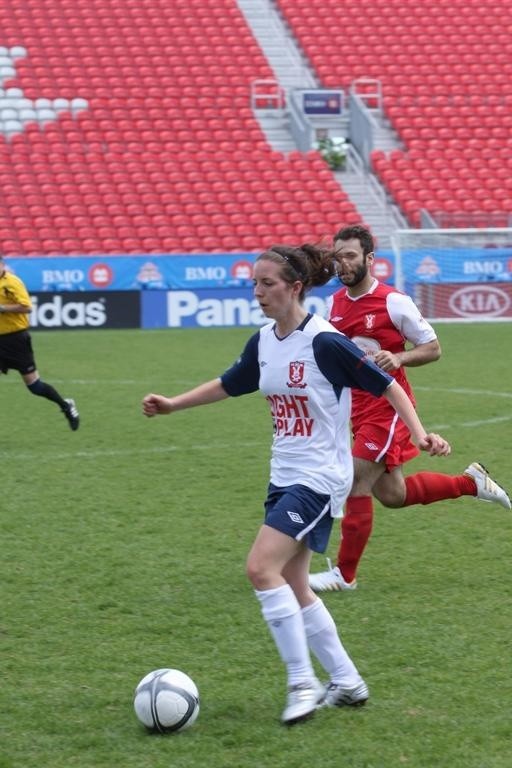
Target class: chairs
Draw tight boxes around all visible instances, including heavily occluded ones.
[1,0,510,261]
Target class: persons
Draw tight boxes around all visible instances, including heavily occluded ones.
[308,224,512,591]
[0,256,79,431]
[143,245,452,725]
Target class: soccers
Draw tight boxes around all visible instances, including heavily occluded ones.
[134,668,199,733]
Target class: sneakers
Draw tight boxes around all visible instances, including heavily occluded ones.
[307,565,360,592]
[317,681,371,707]
[280,679,328,726]
[64,398,80,431]
[463,461,512,513]
